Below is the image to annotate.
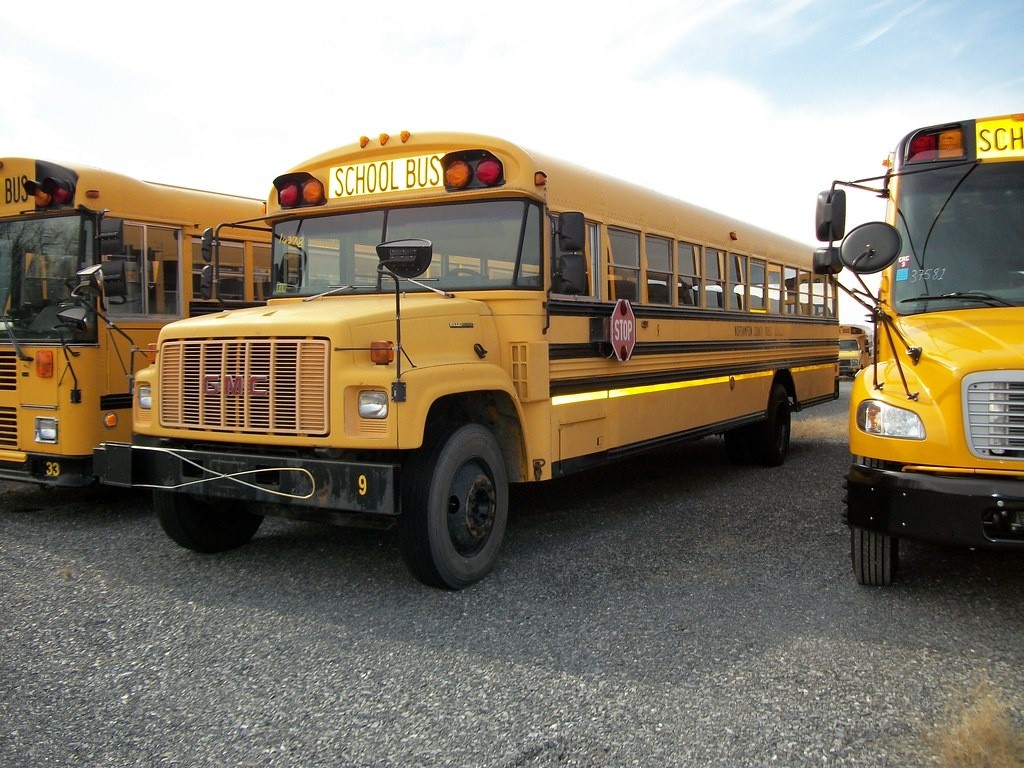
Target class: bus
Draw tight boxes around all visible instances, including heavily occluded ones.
[94,129,843,590]
[1,154,640,496]
[811,112,1022,594]
[836,318,874,383]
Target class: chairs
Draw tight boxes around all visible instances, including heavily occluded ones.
[613,278,832,318]
